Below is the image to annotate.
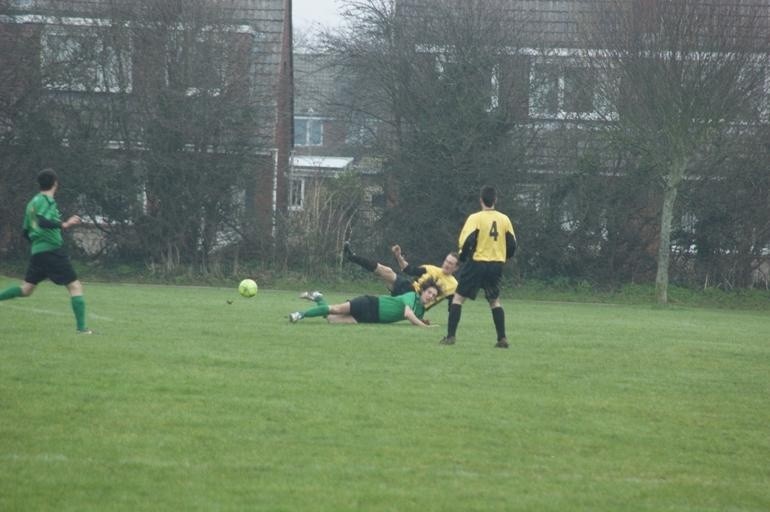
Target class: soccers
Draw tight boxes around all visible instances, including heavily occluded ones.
[238,279,257,298]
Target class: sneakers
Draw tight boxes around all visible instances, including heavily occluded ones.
[299,291,323,301]
[75,327,100,336]
[289,311,302,323]
[494,337,509,349]
[343,240,355,263]
[440,335,456,345]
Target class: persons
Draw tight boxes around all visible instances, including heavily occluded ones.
[439,183,518,349]
[0,168,95,335]
[343,241,463,312]
[290,277,443,328]
